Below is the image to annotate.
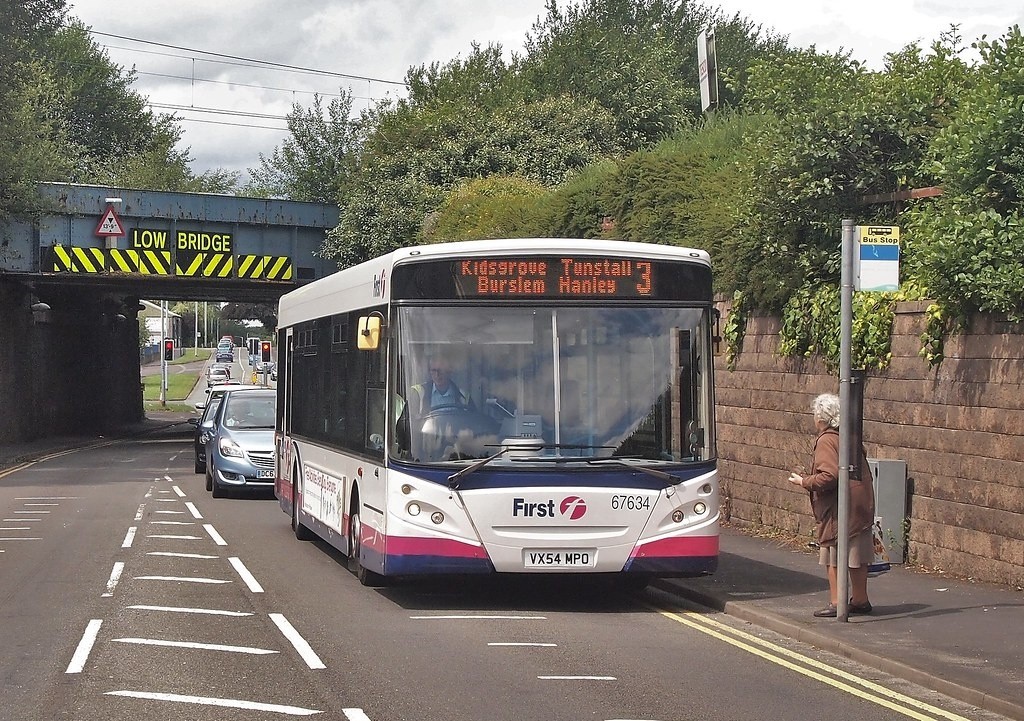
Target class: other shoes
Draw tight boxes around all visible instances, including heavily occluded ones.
[848,596,872,614]
[814,602,849,617]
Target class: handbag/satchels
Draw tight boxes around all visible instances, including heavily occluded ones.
[868,517,891,576]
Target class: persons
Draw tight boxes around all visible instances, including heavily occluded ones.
[227,403,256,426]
[788,393,875,617]
[396,354,477,450]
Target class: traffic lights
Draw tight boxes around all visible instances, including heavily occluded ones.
[163,338,174,362]
[260,340,271,364]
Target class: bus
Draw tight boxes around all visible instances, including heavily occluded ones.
[273,237,722,592]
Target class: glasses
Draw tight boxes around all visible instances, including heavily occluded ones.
[429,368,448,376]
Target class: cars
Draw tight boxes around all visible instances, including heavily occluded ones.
[200,389,276,498]
[187,335,236,474]
[245,336,278,381]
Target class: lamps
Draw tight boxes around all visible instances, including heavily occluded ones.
[32,301,57,324]
[109,312,127,332]
